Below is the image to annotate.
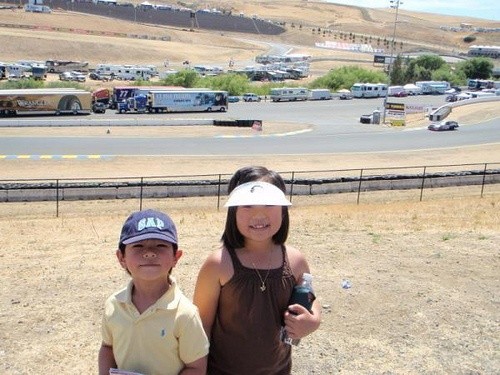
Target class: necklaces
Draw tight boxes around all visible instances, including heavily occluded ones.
[247,242,274,292]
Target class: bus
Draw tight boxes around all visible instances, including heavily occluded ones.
[270,86,308,102]
[350,82,388,98]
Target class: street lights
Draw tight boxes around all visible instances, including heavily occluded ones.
[382,0,404,124]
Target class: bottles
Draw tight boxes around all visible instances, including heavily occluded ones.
[281,273,317,347]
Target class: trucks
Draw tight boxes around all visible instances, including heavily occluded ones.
[0,59,310,83]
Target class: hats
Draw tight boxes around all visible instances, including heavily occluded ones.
[223,181,292,207]
[119,210,178,246]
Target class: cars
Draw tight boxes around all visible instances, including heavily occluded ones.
[228,96,239,103]
[92,102,106,113]
[388,78,500,102]
[427,120,459,131]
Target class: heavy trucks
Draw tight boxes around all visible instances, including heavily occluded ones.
[91,86,159,110]
[0,87,92,116]
[117,90,229,114]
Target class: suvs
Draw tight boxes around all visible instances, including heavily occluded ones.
[242,93,261,102]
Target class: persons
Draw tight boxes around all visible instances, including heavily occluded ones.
[97,208,210,375]
[192,165,321,375]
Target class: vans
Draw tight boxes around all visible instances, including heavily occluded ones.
[309,89,333,100]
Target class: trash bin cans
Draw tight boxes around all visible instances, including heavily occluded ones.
[373,110,380,124]
